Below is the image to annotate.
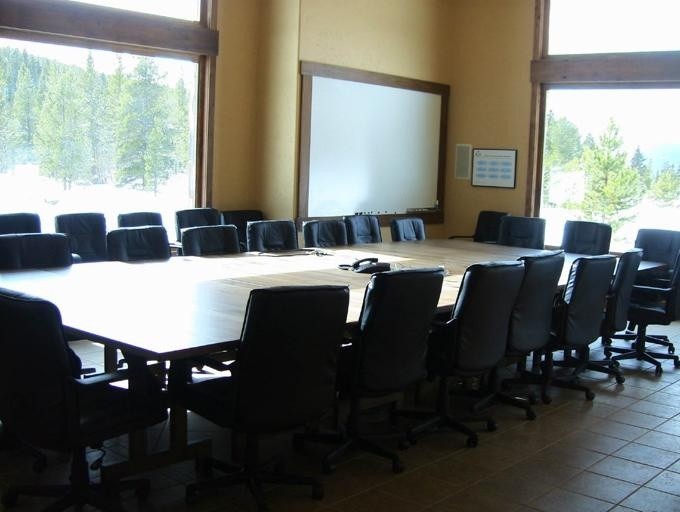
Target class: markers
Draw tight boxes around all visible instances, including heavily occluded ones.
[354,211,374,215]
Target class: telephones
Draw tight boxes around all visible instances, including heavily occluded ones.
[353,258,390,272]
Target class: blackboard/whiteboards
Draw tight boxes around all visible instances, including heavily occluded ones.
[298,60,450,230]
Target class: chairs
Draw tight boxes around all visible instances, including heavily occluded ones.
[0,209,679,512]
